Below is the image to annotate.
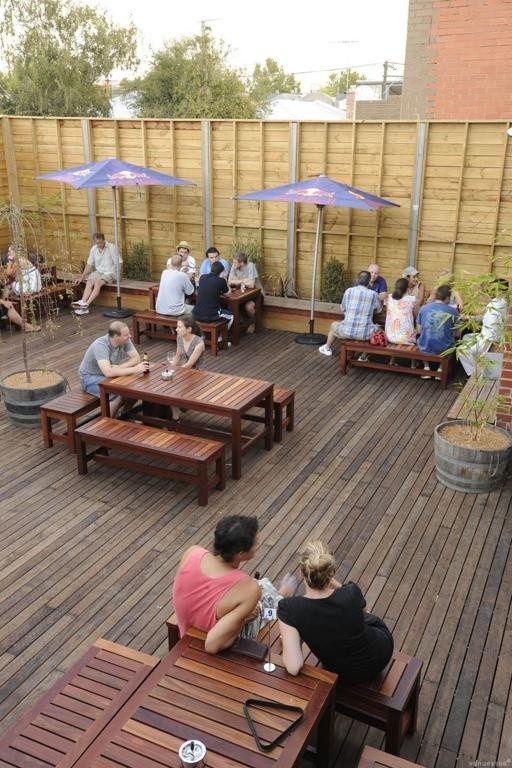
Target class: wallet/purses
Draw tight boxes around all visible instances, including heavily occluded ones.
[231,637,269,661]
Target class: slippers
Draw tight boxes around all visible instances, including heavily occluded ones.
[25,326,41,333]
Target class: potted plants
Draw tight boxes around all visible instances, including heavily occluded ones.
[433,220,512,494]
[0,191,97,428]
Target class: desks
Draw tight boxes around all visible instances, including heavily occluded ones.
[72,626,338,768]
[0,263,57,285]
[148,284,261,345]
[98,361,275,480]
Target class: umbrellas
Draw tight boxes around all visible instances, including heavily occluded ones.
[34,158,196,319]
[227,175,402,345]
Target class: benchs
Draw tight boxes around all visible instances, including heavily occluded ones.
[41,271,345,344]
[166,610,424,757]
[73,416,228,506]
[240,388,296,443]
[0,301,21,331]
[5,283,82,323]
[132,308,229,356]
[339,339,456,390]
[39,387,101,454]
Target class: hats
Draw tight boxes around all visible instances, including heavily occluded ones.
[176,241,192,251]
[402,265,419,277]
[490,278,509,291]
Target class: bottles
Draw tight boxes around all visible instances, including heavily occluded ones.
[456,303,460,313]
[192,276,196,290]
[142,352,150,375]
[240,278,245,294]
[254,571,260,580]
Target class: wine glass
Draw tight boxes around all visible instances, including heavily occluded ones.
[166,352,177,369]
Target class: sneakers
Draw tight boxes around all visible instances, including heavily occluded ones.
[389,361,398,366]
[434,367,442,380]
[358,356,368,362]
[217,337,232,347]
[319,345,333,356]
[246,322,255,334]
[420,367,431,379]
[411,366,416,368]
[71,300,89,309]
[75,308,89,315]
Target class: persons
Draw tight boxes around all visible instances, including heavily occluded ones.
[227,252,265,335]
[425,269,465,313]
[167,240,196,278]
[384,278,421,370]
[198,246,229,287]
[457,278,511,378]
[173,514,298,656]
[78,320,149,418]
[190,262,235,349]
[5,245,42,298]
[318,271,384,356]
[70,232,123,315]
[155,255,195,316]
[353,263,388,306]
[415,285,461,381]
[277,542,394,686]
[401,266,425,334]
[0,275,43,332]
[170,316,206,425]
